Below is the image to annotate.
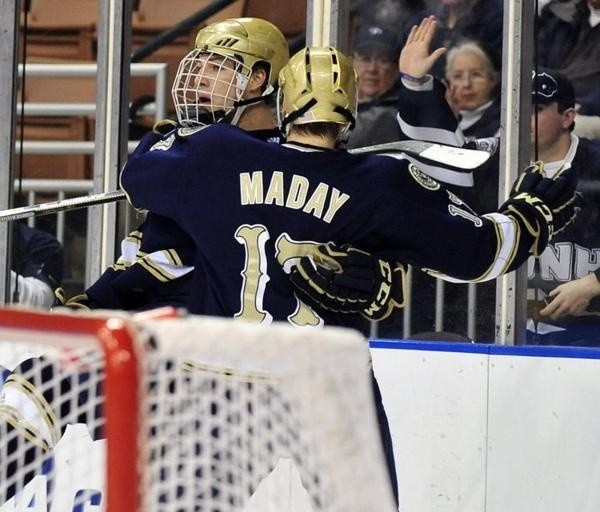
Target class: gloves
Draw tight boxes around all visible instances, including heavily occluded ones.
[152,106,204,133]
[286,239,411,325]
[500,157,576,274]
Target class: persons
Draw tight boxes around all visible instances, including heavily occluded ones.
[118,40,579,512]
[5,213,77,506]
[331,1,600,348]
[66,14,406,512]
[122,82,159,143]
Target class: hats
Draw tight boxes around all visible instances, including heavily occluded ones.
[353,24,398,61]
[531,66,577,106]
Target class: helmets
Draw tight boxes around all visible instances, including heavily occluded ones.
[171,13,293,128]
[277,43,361,140]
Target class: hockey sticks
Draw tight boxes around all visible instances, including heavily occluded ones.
[0,140,490,223]
